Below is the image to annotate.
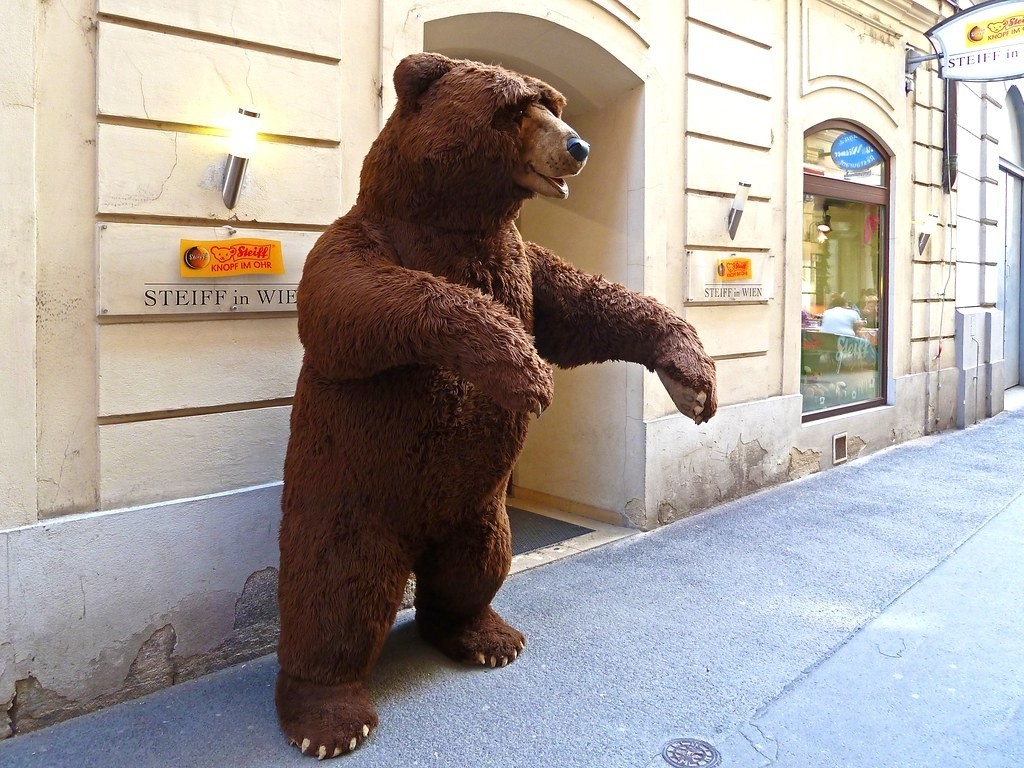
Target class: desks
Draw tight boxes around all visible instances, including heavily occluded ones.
[855,326,879,348]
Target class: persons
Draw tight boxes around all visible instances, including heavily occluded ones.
[830,288,878,328]
[821,298,875,370]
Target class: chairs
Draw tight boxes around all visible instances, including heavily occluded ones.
[801,328,876,375]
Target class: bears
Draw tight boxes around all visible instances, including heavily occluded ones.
[274,49,716,762]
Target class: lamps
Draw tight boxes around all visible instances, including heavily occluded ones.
[918,212,938,256]
[807,221,828,244]
[220,108,262,209]
[817,202,832,232]
[727,181,752,240]
